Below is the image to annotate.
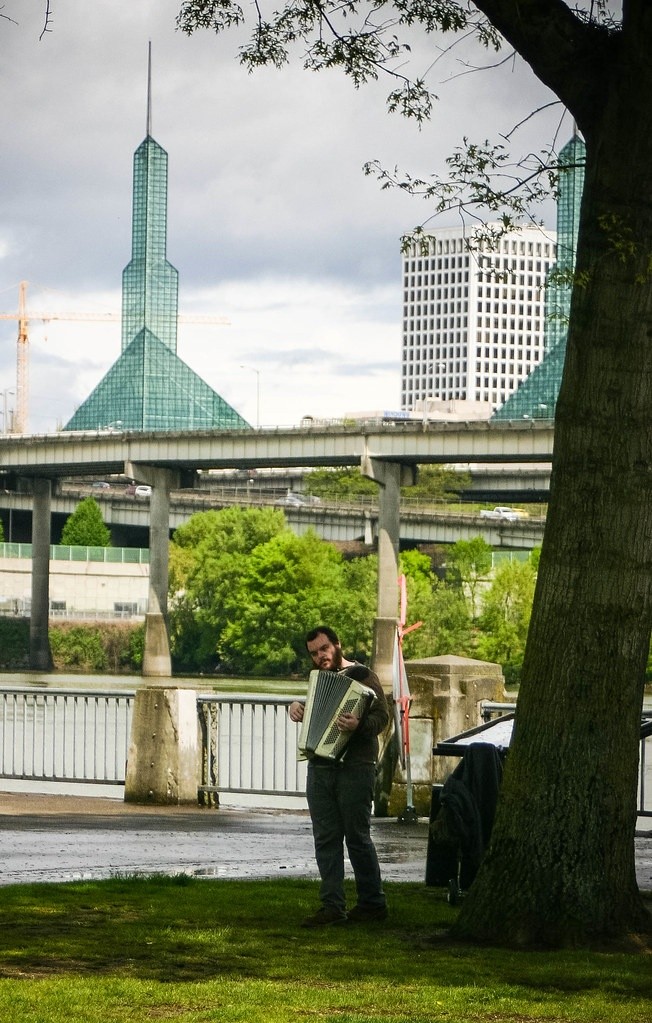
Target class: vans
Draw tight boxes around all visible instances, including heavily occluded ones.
[135,485,151,496]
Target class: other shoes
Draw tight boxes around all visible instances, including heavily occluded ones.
[304,907,348,926]
[346,902,388,920]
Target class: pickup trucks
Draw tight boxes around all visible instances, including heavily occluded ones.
[480,506,520,520]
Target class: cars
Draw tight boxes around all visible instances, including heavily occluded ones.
[93,482,110,489]
[274,495,305,507]
[125,485,137,494]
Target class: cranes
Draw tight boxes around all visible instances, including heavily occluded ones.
[0,281,232,433]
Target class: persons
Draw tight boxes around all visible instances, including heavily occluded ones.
[290,627,390,924]
